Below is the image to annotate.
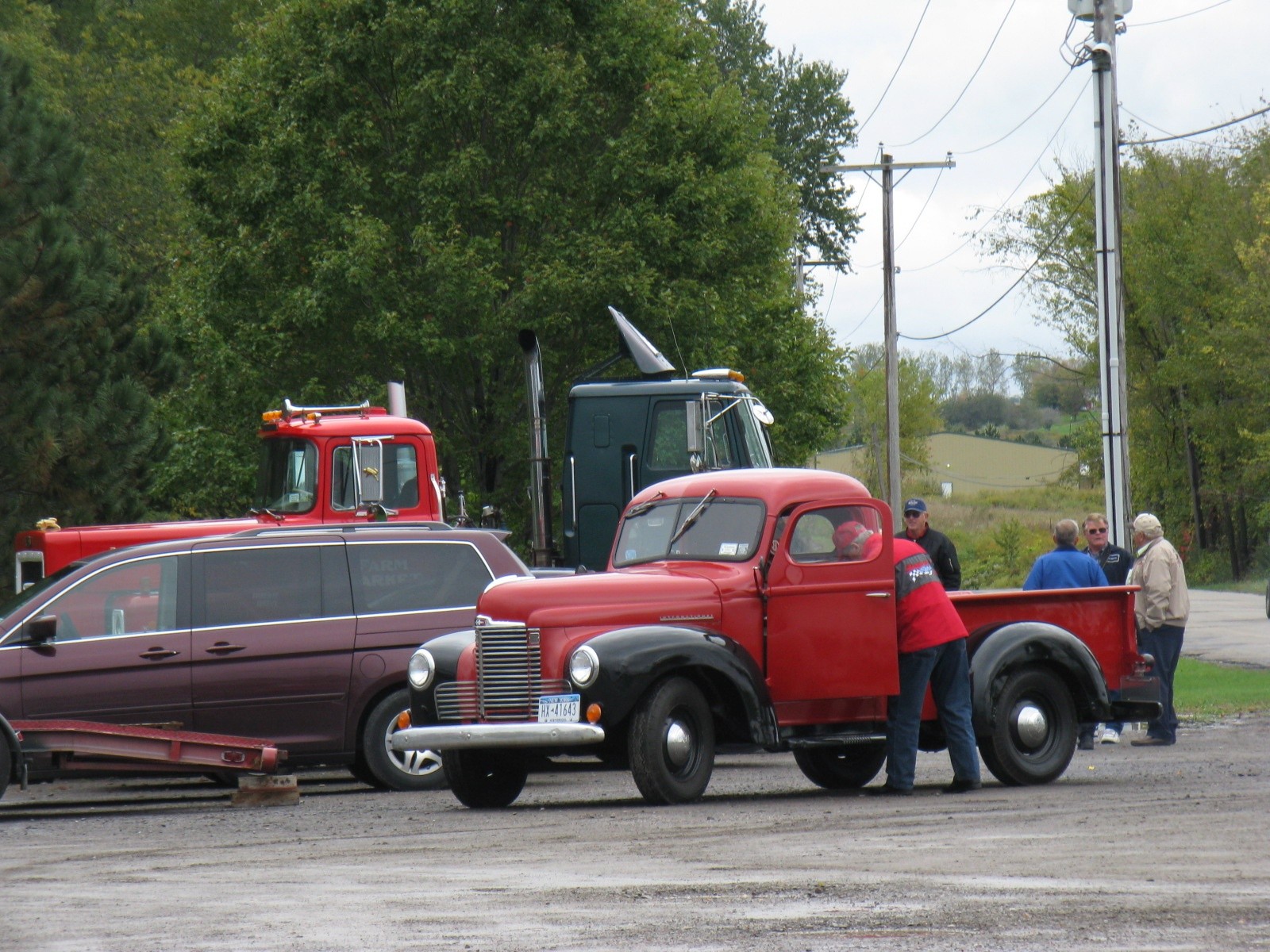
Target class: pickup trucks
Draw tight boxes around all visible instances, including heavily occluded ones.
[390,467,1164,808]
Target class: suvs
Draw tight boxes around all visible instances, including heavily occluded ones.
[0,521,534,793]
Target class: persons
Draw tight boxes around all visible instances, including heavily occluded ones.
[833,520,982,796]
[1126,513,1190,746]
[1022,519,1109,749]
[1079,513,1135,744]
[894,498,961,591]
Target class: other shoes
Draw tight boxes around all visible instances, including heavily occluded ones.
[868,782,913,796]
[1131,735,1170,747]
[1101,729,1120,744]
[942,777,982,794]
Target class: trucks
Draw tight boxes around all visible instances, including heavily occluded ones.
[10,303,807,644]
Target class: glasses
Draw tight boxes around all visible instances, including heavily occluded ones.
[1089,528,1106,535]
[904,511,921,518]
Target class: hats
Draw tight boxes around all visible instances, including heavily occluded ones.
[904,499,927,512]
[1126,513,1161,532]
[831,521,868,557]
